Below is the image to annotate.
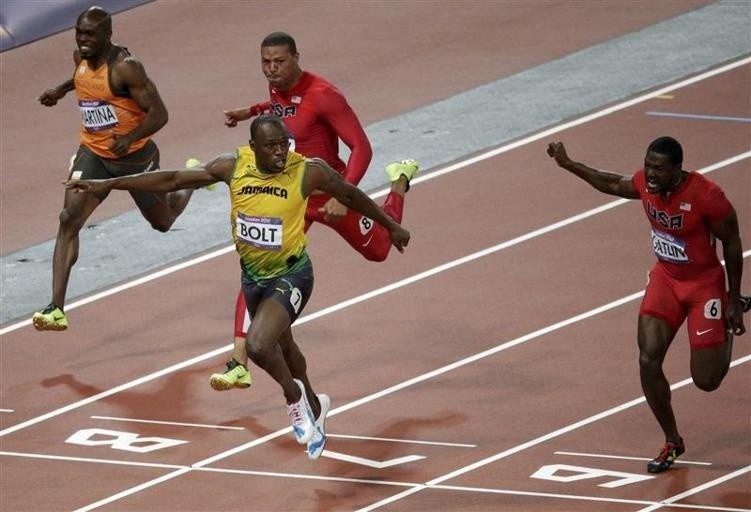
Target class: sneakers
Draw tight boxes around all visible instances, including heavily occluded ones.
[385,159,419,192]
[33,303,68,330]
[284,378,316,445]
[648,437,685,473]
[186,159,217,192]
[209,357,252,391]
[740,296,751,312]
[306,393,330,460]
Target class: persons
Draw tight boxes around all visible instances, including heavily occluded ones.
[56,113,411,465]
[31,4,216,332]
[207,31,420,392]
[544,132,751,476]
